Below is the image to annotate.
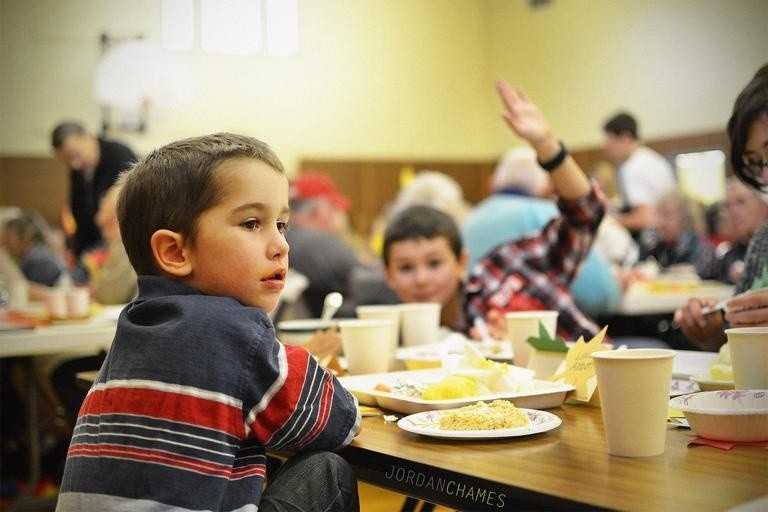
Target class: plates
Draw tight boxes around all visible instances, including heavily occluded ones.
[672,388,768,443]
[670,345,737,389]
[396,408,562,441]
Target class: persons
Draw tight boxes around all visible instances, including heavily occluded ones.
[2,121,142,290]
[671,63,768,353]
[54,133,361,511]
[380,79,610,344]
[463,114,768,354]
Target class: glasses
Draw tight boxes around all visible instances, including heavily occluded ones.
[743,152,768,172]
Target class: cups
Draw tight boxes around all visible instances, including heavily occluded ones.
[276,300,560,376]
[725,326,768,390]
[7,280,91,320]
[589,348,677,459]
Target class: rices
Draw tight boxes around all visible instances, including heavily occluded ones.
[438,399,528,430]
